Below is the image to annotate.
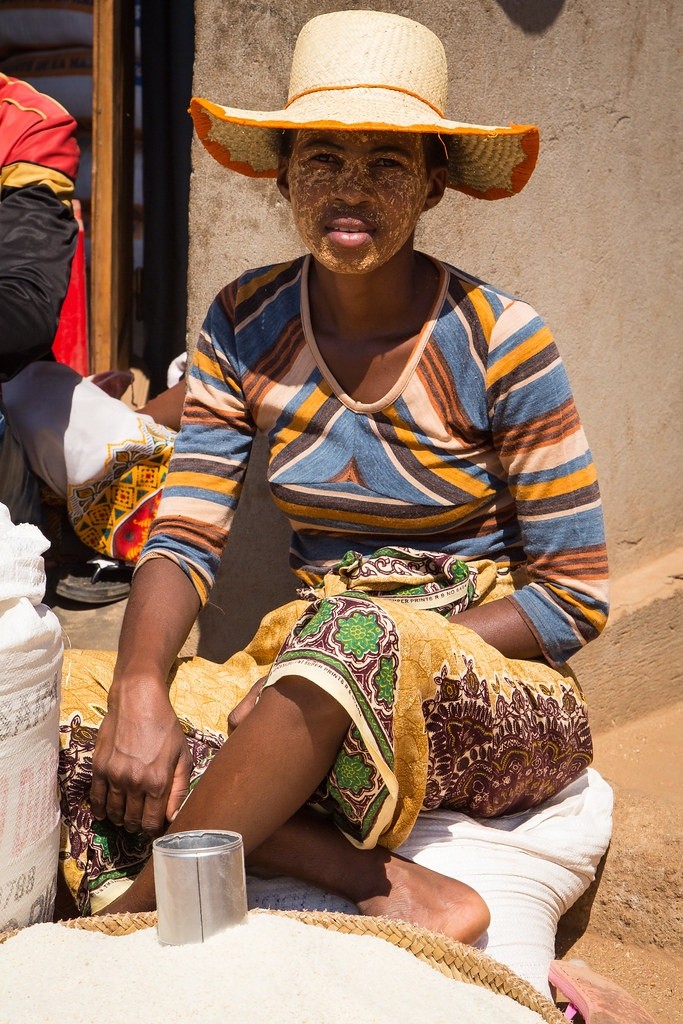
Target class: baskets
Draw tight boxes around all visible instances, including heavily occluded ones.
[0,907,574,1024]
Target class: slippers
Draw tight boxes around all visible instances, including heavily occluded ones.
[55,553,132,603]
[548,960,658,1024]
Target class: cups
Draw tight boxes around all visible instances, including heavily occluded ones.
[152,830,249,946]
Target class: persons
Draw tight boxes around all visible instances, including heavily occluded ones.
[0,69,187,609]
[51,11,612,950]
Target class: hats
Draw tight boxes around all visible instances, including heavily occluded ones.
[191,10,540,200]
